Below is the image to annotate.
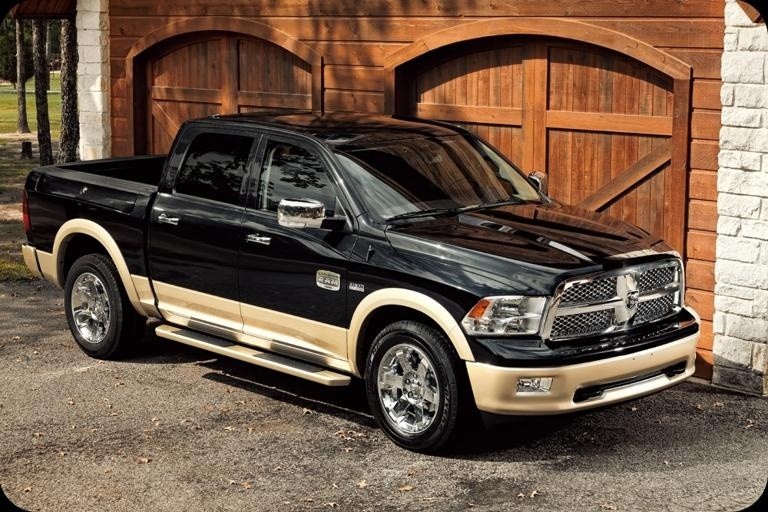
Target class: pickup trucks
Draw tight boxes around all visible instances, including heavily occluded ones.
[19,109,702,449]
